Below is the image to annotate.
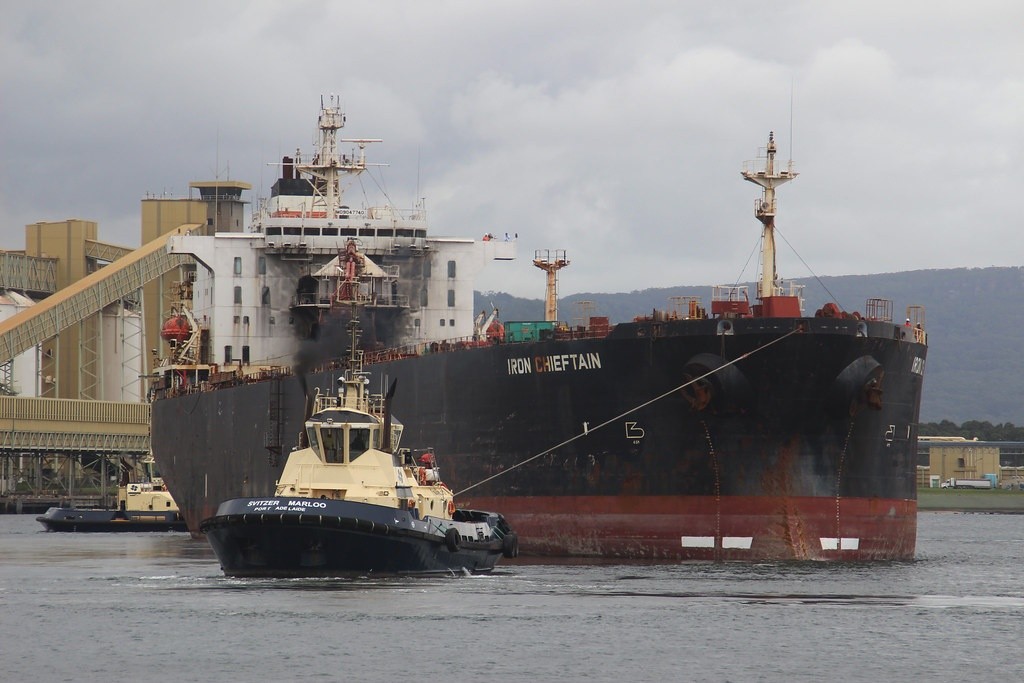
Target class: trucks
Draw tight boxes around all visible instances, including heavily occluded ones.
[941,477,993,490]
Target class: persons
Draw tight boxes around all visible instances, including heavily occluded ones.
[915,322,924,342]
[295,148,302,164]
[482,232,510,242]
[904,318,911,327]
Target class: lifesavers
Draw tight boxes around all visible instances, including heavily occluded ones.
[445,528,462,553]
[502,531,519,559]
[448,501,456,515]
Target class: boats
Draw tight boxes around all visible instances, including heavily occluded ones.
[35,477,190,532]
[161,316,188,339]
[197,299,519,577]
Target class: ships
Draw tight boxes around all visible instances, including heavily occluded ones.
[136,91,932,565]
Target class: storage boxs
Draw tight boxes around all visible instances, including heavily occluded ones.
[712,301,749,315]
[590,317,608,338]
[503,320,557,343]
